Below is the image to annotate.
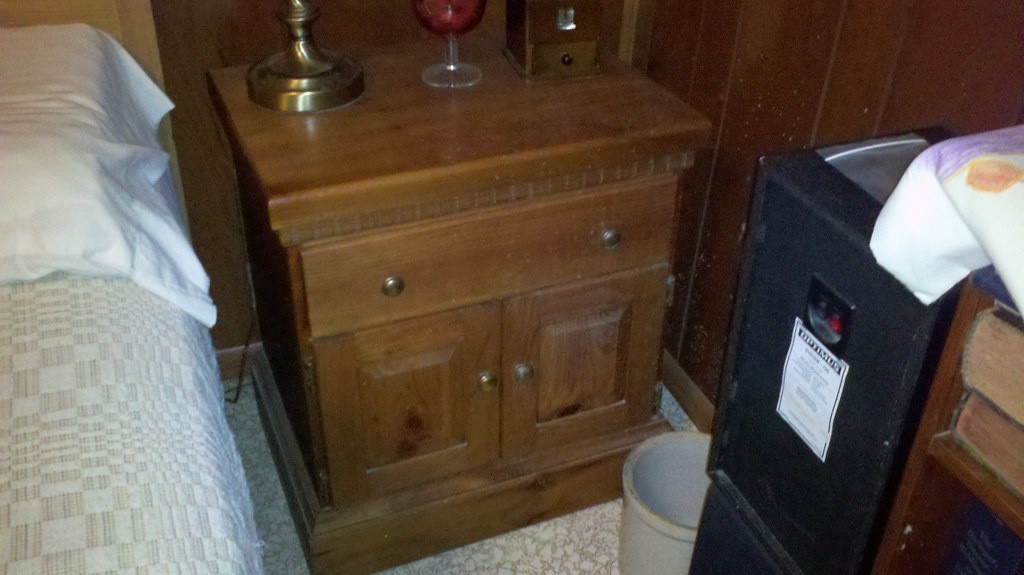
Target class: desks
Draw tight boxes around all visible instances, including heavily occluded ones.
[868,264,1024,575]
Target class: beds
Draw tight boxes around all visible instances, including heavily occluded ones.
[0,21,266,575]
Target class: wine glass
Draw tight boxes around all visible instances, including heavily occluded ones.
[412,0,488,89]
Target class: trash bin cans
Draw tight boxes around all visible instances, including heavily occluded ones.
[614,432,711,574]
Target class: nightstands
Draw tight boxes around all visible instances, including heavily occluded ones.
[206,38,715,575]
